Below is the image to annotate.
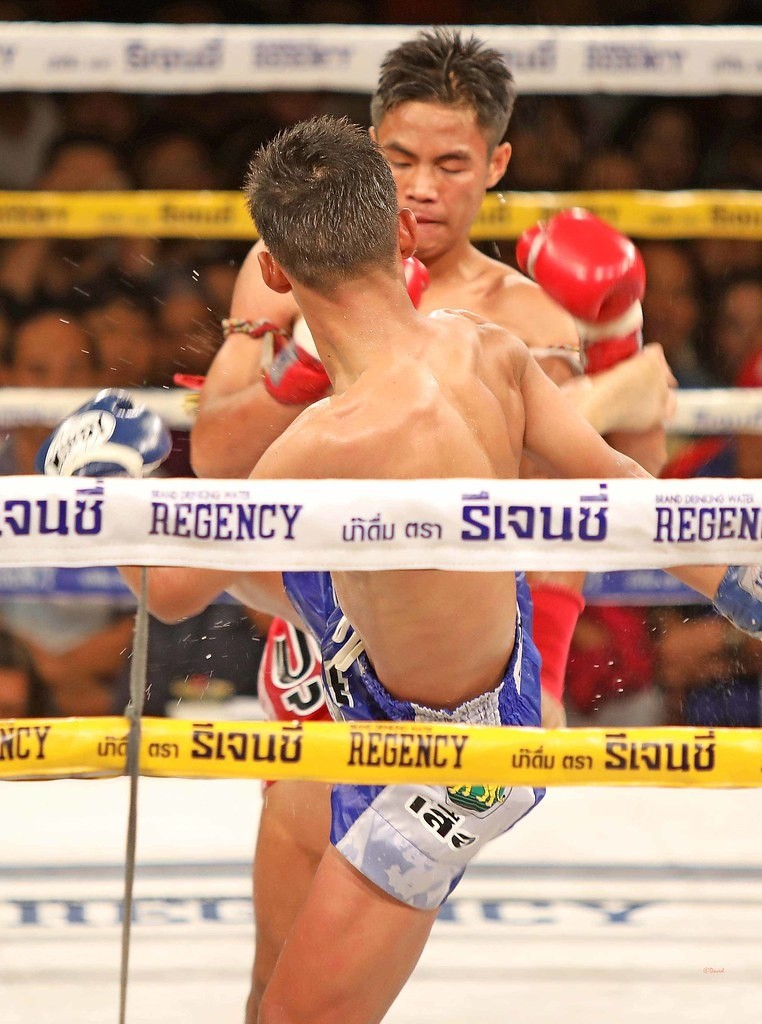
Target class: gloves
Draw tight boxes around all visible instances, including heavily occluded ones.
[266,254,432,403]
[36,387,173,477]
[515,205,650,375]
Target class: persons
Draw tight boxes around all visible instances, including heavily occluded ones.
[0,93,762,725]
[46,121,762,1024]
[188,34,670,1024]
